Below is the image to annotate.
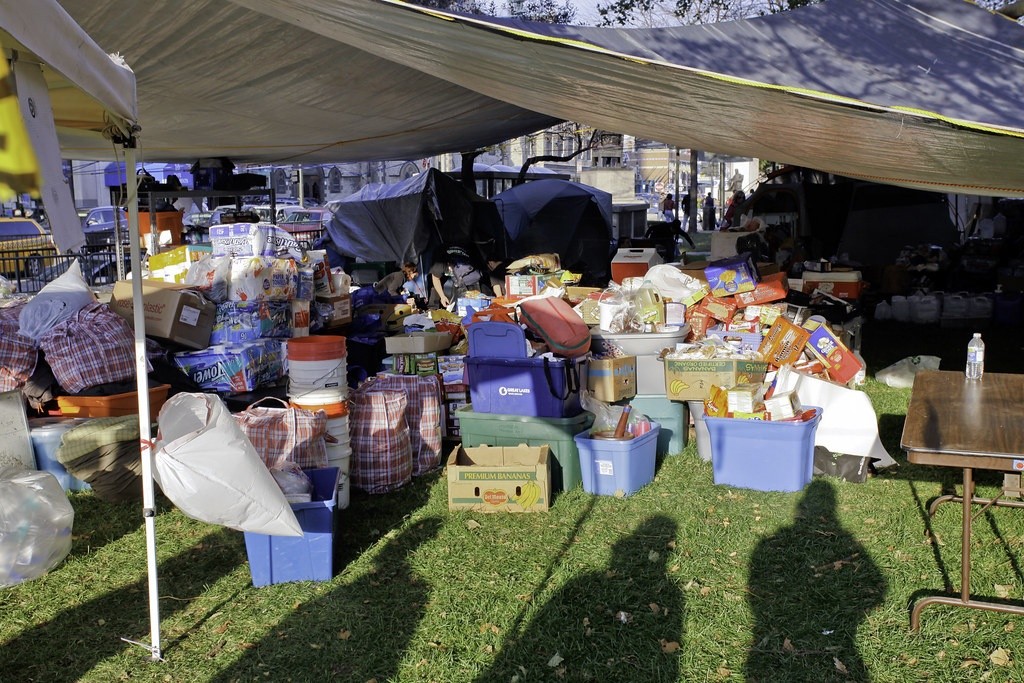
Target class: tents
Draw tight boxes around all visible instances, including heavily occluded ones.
[0,1,161,659]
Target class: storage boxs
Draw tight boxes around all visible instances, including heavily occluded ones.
[29,385,171,493]
[125,212,184,246]
[244,233,897,588]
[945,251,981,287]
[110,279,218,349]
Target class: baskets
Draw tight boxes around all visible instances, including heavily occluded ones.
[580,300,599,325]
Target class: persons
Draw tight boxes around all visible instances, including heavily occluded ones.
[727,190,737,206]
[682,189,690,230]
[705,192,719,227]
[373,243,513,311]
[644,219,695,262]
[729,169,744,194]
[658,193,674,221]
[609,235,631,259]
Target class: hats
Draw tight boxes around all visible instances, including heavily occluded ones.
[430,250,446,277]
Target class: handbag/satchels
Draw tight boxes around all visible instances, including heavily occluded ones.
[0,300,39,395]
[874,355,941,388]
[351,387,412,494]
[232,396,329,469]
[185,254,231,303]
[39,302,153,394]
[365,375,443,476]
[515,296,592,400]
[659,202,664,211]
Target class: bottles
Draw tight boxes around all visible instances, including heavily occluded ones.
[966,332,985,380]
[638,280,665,324]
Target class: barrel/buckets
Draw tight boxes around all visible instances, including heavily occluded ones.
[598,301,622,332]
[286,335,353,510]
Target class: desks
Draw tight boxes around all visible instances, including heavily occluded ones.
[900,371,1024,632]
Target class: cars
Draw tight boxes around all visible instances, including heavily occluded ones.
[1,216,57,278]
[185,203,327,242]
[85,205,131,231]
